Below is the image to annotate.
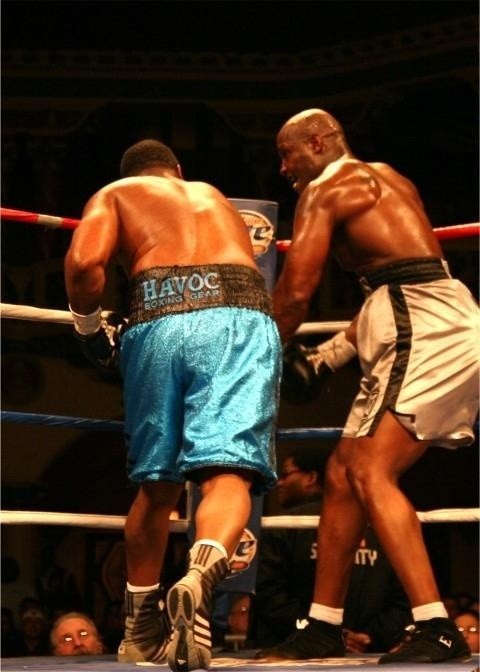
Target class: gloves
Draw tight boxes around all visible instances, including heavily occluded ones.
[279,329,358,401]
[65,303,129,375]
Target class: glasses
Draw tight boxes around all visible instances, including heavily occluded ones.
[280,469,304,481]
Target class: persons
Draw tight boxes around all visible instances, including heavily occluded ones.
[265,106,479,666]
[62,136,283,668]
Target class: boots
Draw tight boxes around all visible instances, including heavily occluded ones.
[115,579,177,667]
[162,540,234,671]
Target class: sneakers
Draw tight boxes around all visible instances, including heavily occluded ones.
[377,615,474,667]
[252,615,348,657]
